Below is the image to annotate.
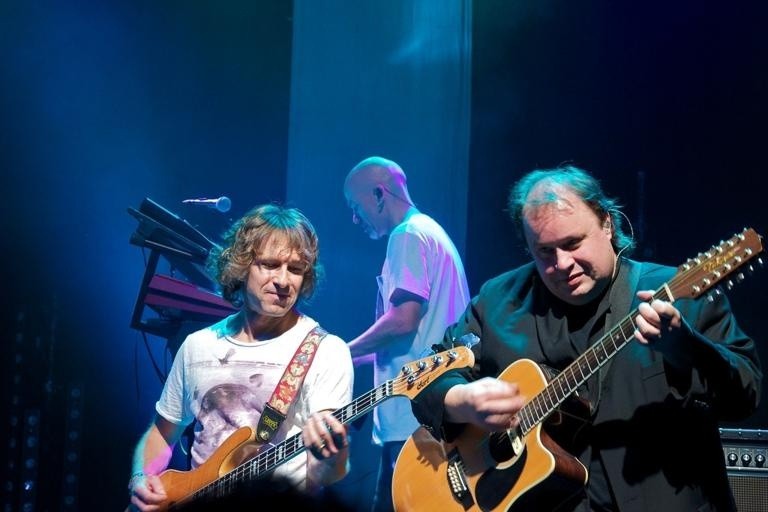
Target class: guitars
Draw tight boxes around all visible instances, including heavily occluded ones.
[128,337,476,512]
[391,227,762,512]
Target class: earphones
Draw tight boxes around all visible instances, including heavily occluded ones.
[603,215,610,228]
[375,186,382,196]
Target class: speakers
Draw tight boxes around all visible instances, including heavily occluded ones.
[719,427,768,511]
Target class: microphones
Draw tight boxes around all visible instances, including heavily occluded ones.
[182,196,232,213]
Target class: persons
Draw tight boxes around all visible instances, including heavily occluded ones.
[128,202,355,512]
[409,158,763,512]
[345,158,471,511]
